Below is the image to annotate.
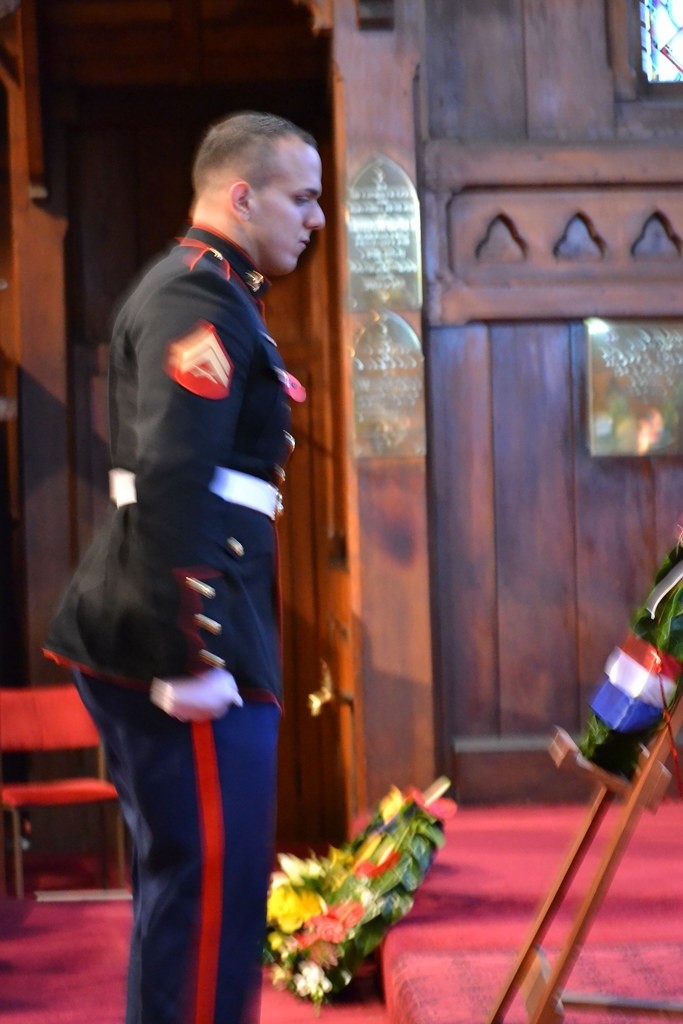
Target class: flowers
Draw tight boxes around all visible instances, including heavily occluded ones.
[261,778,459,1013]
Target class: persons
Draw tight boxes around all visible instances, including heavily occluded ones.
[40,114,325,1024]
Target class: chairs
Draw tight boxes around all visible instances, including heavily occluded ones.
[0,684,127,901]
